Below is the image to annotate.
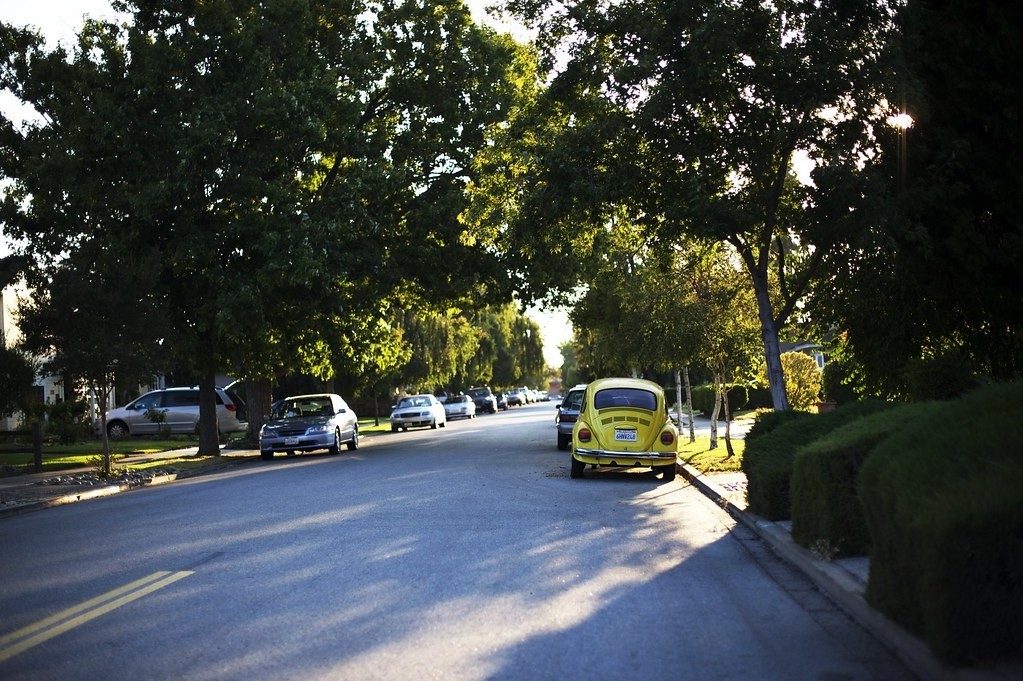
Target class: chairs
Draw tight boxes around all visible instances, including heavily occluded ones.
[631,395,652,410]
[597,393,614,408]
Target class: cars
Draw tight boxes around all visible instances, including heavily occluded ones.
[519,388,551,405]
[507,388,527,407]
[443,395,477,421]
[570,377,678,482]
[493,390,508,411]
[555,383,630,451]
[390,395,447,432]
[259,394,359,461]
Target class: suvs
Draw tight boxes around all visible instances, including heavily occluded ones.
[466,384,498,413]
[92,369,263,444]
